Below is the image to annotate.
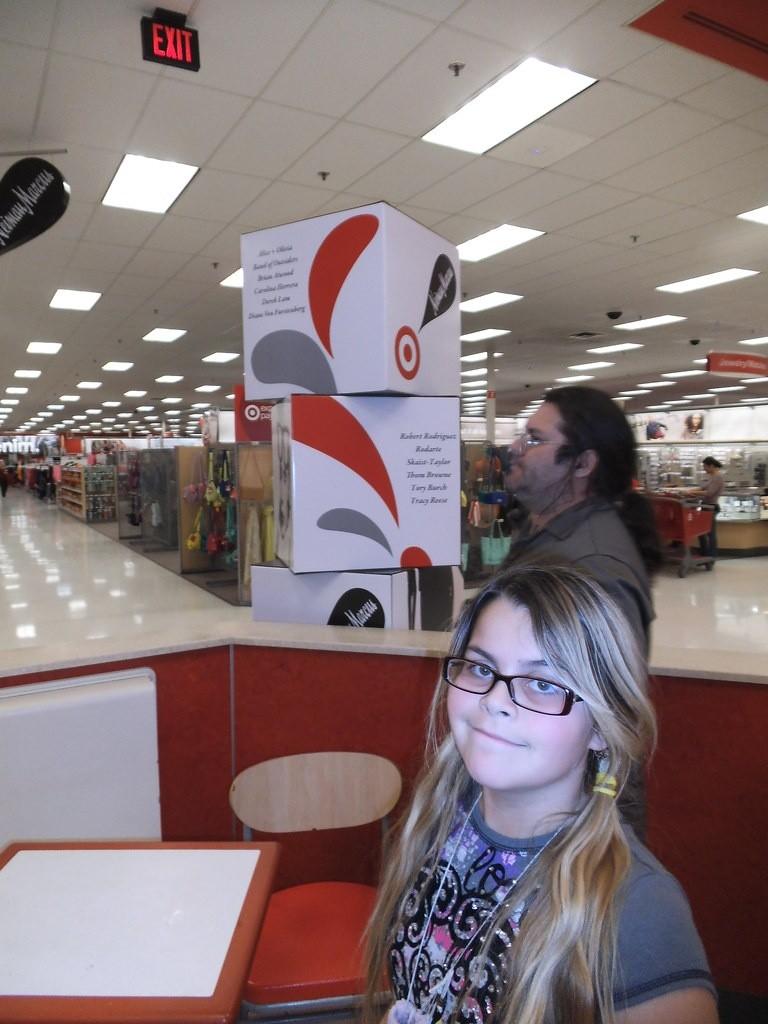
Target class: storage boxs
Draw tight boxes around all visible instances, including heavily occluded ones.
[250,559,465,633]
[271,393,461,575]
[239,199,461,402]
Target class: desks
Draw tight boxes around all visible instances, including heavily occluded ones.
[0,840,283,1024]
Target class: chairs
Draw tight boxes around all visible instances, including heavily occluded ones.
[227,750,403,1024]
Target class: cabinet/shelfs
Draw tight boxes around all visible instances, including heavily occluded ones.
[56,464,119,524]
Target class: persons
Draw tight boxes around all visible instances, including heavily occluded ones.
[350,559,721,1024]
[499,388,658,666]
[688,457,725,571]
[646,415,669,440]
[681,412,705,440]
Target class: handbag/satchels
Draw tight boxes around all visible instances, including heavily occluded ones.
[129,495,140,525]
[462,441,509,529]
[461,543,470,571]
[185,451,238,567]
[480,519,512,565]
[129,457,140,488]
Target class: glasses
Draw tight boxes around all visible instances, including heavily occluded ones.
[520,427,573,449]
[443,656,584,716]
